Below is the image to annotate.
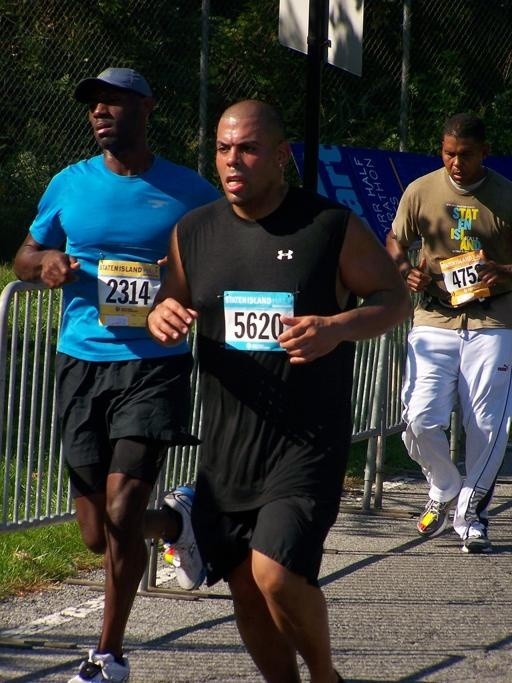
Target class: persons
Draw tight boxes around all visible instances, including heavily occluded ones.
[149,98,415,683]
[14,67,227,683]
[384,110,511,554]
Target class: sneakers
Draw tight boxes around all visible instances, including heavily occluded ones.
[463,515,492,551]
[414,486,461,538]
[67,649,132,683]
[161,483,209,595]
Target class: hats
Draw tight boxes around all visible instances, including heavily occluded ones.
[71,62,157,103]
